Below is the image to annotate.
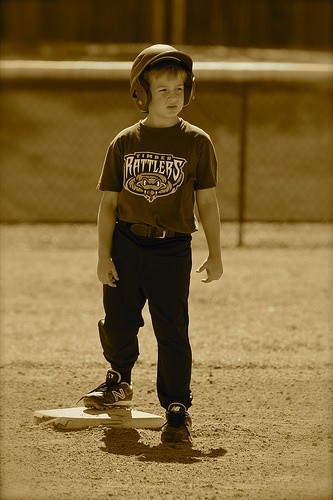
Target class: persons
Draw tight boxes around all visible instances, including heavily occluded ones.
[83,44,223,441]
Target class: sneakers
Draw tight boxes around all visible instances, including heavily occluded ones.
[83,367,134,411]
[160,402,193,446]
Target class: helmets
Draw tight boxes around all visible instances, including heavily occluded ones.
[129,44,196,114]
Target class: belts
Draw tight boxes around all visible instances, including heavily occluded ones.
[117,221,184,240]
